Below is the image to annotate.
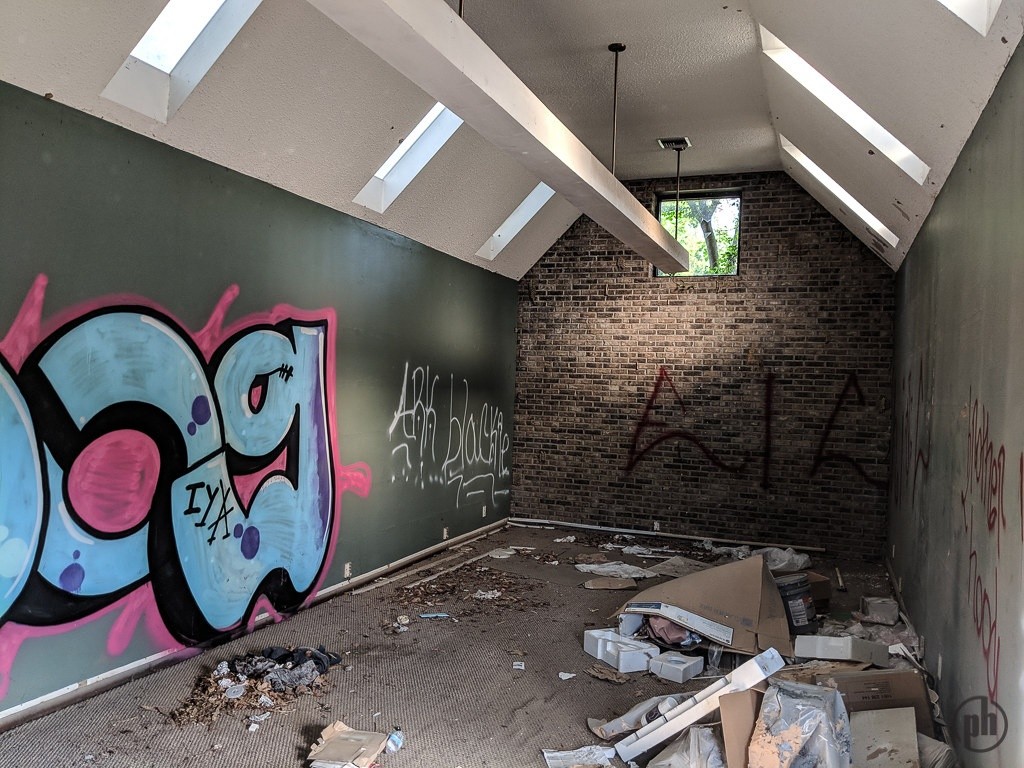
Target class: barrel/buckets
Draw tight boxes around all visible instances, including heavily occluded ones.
[774,572,818,636]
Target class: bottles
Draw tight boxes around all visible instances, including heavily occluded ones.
[385,731,406,755]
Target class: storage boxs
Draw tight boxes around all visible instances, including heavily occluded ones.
[606,555,794,658]
